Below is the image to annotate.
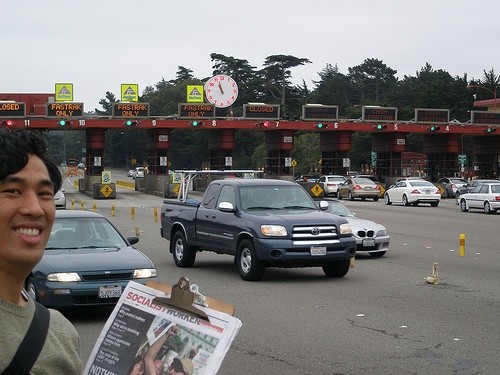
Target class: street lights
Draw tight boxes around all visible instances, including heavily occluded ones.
[466,85,497,123]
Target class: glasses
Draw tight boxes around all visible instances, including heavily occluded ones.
[169,363,185,375]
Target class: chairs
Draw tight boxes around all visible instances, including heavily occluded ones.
[55,229,74,243]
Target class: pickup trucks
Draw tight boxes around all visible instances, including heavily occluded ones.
[159,179,357,282]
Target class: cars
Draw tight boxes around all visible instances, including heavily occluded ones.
[317,174,348,198]
[289,198,391,258]
[127,167,145,180]
[336,178,380,202]
[384,180,441,207]
[436,177,500,205]
[393,177,425,186]
[459,183,500,215]
[54,189,67,209]
[61,159,85,177]
[350,174,386,198]
[294,173,321,183]
[22,209,160,318]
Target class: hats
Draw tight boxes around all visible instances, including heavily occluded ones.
[173,357,194,375]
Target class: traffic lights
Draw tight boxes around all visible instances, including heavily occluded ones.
[57,120,73,126]
[256,122,270,128]
[190,121,204,127]
[124,120,138,126]
[426,125,440,131]
[315,123,329,129]
[0,120,15,127]
[372,124,387,130]
[482,127,496,133]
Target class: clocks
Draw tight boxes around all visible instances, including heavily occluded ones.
[205,75,238,108]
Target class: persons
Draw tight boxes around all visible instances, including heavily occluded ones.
[131,326,195,375]
[0,128,85,375]
